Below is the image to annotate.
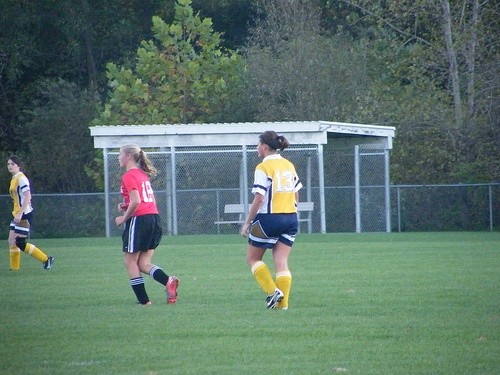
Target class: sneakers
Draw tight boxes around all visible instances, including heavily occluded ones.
[283,307,287,309]
[166,276,179,304]
[136,302,152,305]
[43,256,54,270]
[264,288,284,310]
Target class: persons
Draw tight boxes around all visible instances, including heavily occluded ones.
[241,131,302,310]
[115,144,179,305]
[7,157,55,271]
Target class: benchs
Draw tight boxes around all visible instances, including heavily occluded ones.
[214,201,314,234]
